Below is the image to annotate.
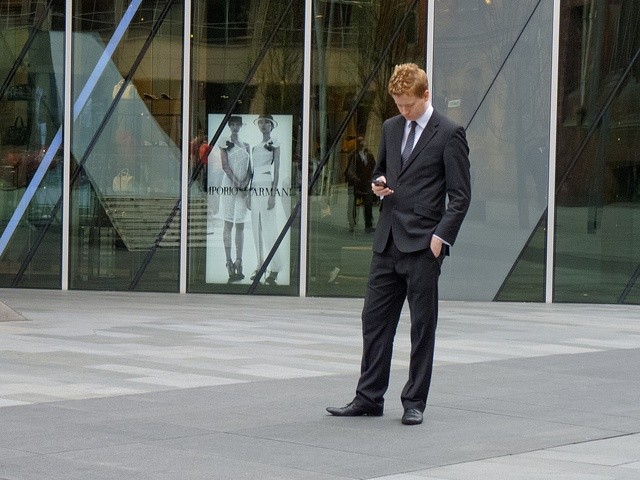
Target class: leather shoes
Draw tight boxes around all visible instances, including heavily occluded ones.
[326,401,383,416]
[402,408,423,425]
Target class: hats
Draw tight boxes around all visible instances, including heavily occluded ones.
[228,116,242,126]
[253,115,278,128]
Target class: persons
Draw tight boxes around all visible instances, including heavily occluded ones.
[324,62,471,425]
[217,116,251,280]
[345,136,378,233]
[247,115,291,282]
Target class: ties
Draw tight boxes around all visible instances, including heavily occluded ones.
[401,121,418,171]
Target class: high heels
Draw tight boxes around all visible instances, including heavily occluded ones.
[226,259,238,279]
[233,258,245,279]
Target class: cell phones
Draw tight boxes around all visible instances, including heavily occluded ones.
[373,180,391,189]
